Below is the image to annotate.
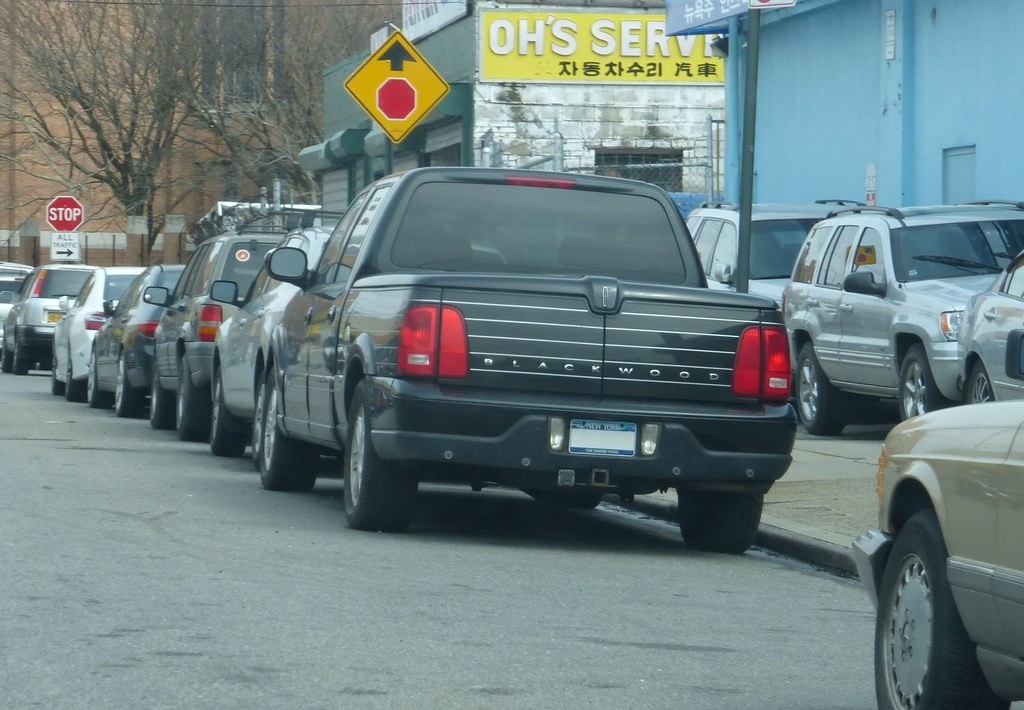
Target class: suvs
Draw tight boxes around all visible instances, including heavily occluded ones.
[142,200,346,443]
[683,196,865,316]
[780,200,1024,437]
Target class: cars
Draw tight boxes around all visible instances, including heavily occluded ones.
[0,264,117,375]
[83,263,261,417]
[47,266,153,401]
[208,222,507,474]
[0,262,41,358]
[954,250,1024,405]
[850,399,1024,709]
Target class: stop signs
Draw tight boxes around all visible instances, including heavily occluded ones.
[47,196,84,234]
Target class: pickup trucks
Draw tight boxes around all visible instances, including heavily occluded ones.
[260,166,797,555]
